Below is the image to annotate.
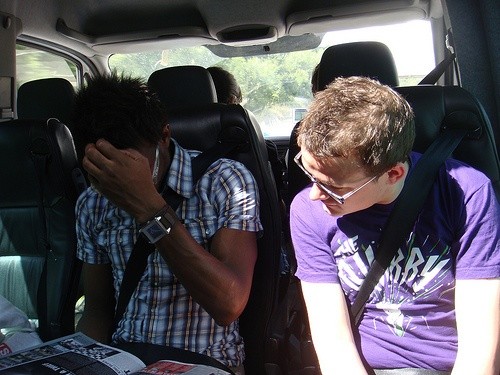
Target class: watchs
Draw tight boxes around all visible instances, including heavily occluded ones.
[139,206,179,244]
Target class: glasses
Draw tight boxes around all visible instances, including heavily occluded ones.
[294,150,406,205]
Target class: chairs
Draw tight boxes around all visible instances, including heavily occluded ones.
[0,42,500,375]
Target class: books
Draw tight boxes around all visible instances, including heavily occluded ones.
[0,332,231,375]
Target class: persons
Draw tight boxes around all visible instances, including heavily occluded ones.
[287,64,319,183]
[290,76,500,375]
[69,74,263,375]
[210,66,285,199]
